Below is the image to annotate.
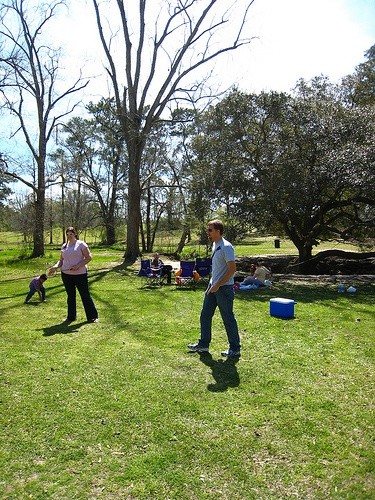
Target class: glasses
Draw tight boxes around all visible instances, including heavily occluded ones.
[209,228,212,232]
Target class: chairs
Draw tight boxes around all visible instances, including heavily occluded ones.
[137,257,212,291]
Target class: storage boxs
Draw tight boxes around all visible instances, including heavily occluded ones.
[270,297,297,319]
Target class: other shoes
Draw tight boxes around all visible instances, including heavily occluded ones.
[66,319,73,324]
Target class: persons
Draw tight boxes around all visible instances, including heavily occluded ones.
[235,258,273,288]
[151,252,172,285]
[187,219,242,357]
[48,226,100,323]
[23,274,48,305]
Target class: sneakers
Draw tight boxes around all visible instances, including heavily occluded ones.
[221,349,241,357]
[188,339,209,352]
